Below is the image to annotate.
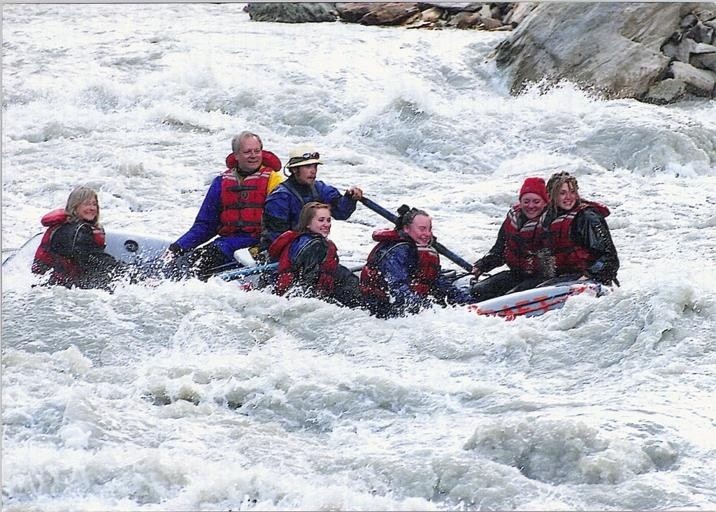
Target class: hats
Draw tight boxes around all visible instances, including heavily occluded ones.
[519,176,549,203]
[283,144,324,168]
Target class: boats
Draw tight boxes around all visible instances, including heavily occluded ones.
[1,231,610,322]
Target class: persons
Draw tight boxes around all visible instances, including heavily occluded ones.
[471,177,552,305]
[359,208,475,319]
[160,129,287,275]
[255,143,364,271]
[265,201,358,307]
[505,170,620,294]
[29,185,128,290]
